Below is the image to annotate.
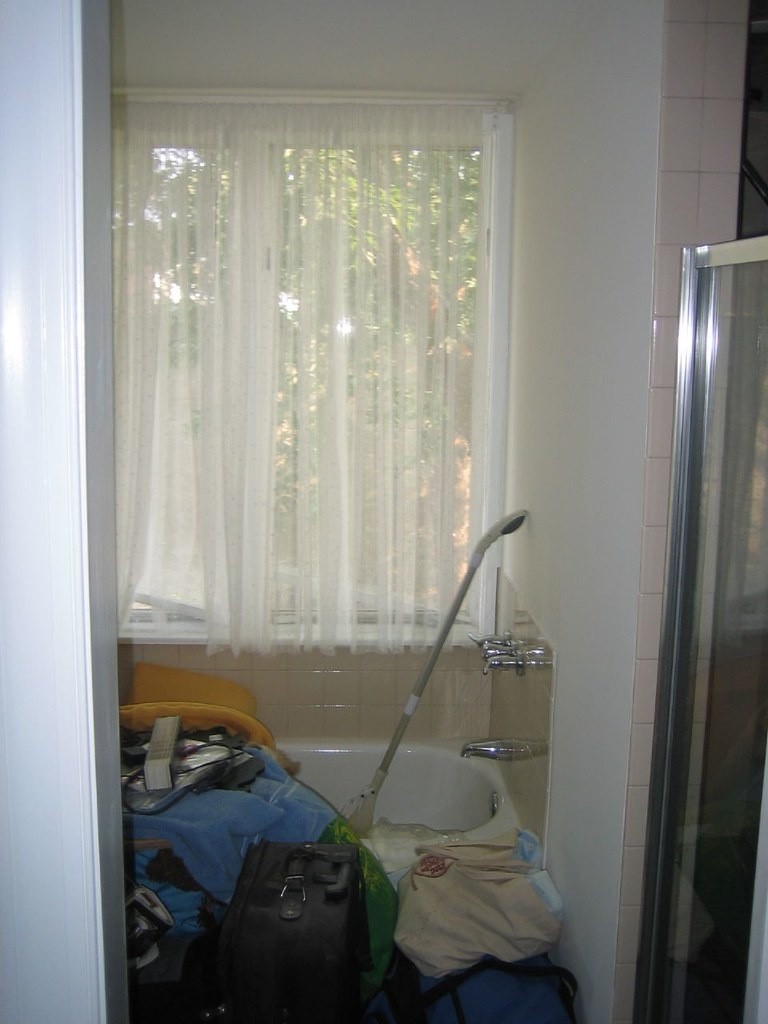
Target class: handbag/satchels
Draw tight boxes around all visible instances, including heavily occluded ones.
[393,838,566,979]
[415,952,577,1024]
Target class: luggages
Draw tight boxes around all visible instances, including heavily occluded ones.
[216,840,364,1024]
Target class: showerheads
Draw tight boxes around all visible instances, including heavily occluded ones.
[476,510,527,556]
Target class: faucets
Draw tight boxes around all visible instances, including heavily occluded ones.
[460,738,513,761]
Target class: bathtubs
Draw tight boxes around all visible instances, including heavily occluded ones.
[273,741,520,841]
